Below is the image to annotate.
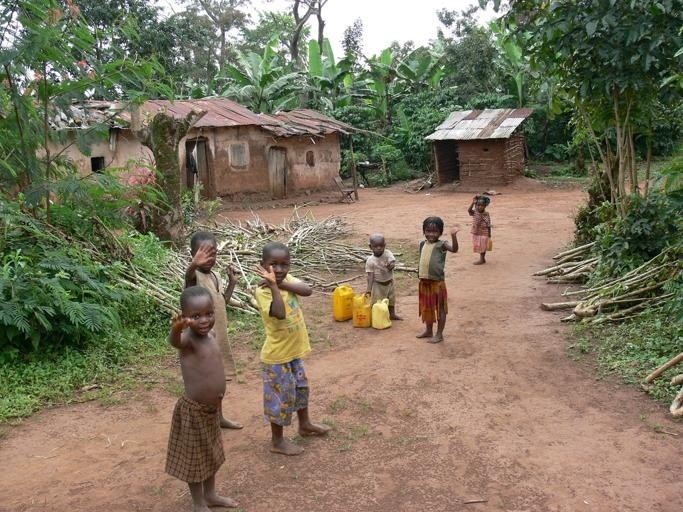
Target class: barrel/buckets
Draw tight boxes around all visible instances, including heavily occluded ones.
[372,298,392,329]
[352,294,371,327]
[333,285,353,321]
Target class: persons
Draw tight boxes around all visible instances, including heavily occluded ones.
[252,242,332,455]
[364,233,405,321]
[184,230,244,430]
[414,215,461,344]
[468,195,493,265]
[163,285,242,512]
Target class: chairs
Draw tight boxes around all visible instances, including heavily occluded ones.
[334,175,354,204]
